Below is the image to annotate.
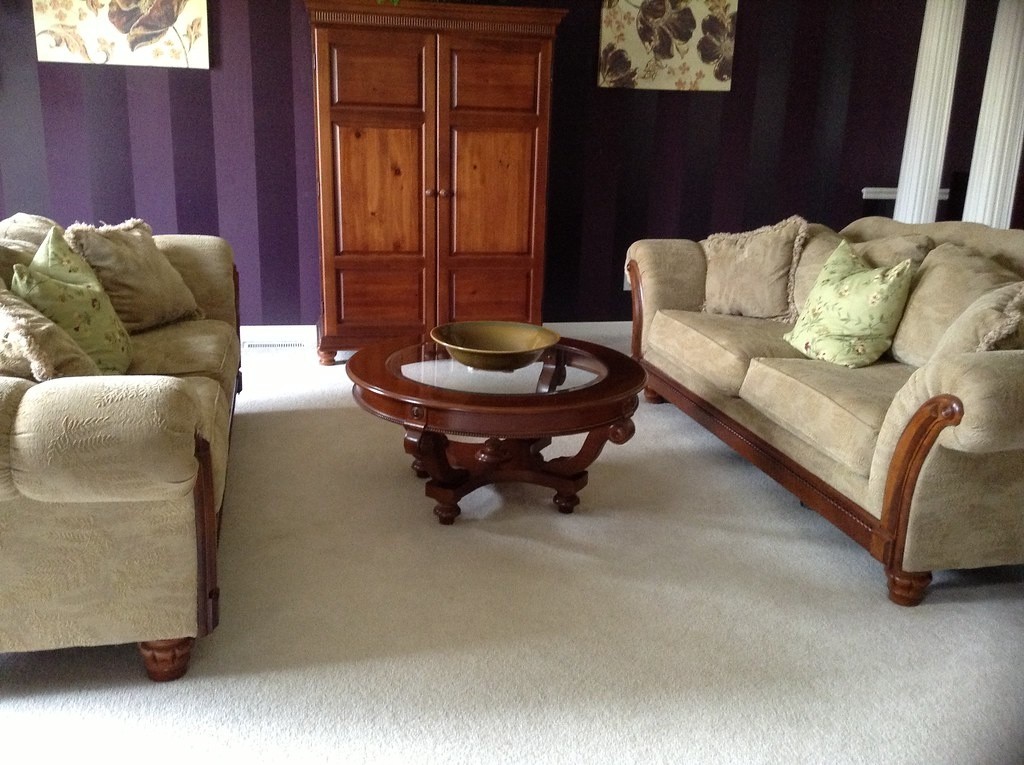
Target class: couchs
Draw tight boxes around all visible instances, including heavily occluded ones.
[0,211,242,684]
[623,216,1024,607]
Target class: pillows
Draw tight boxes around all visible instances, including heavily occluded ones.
[788,223,936,325]
[885,241,1024,367]
[930,280,1024,353]
[0,210,65,288]
[701,214,808,324]
[10,224,136,375]
[783,239,913,370]
[63,215,207,333]
[0,287,102,383]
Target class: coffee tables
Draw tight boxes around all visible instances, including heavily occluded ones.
[346,336,649,525]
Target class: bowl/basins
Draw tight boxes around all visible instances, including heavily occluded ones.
[430,321,561,370]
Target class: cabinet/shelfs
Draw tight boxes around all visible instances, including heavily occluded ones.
[303,0,570,366]
